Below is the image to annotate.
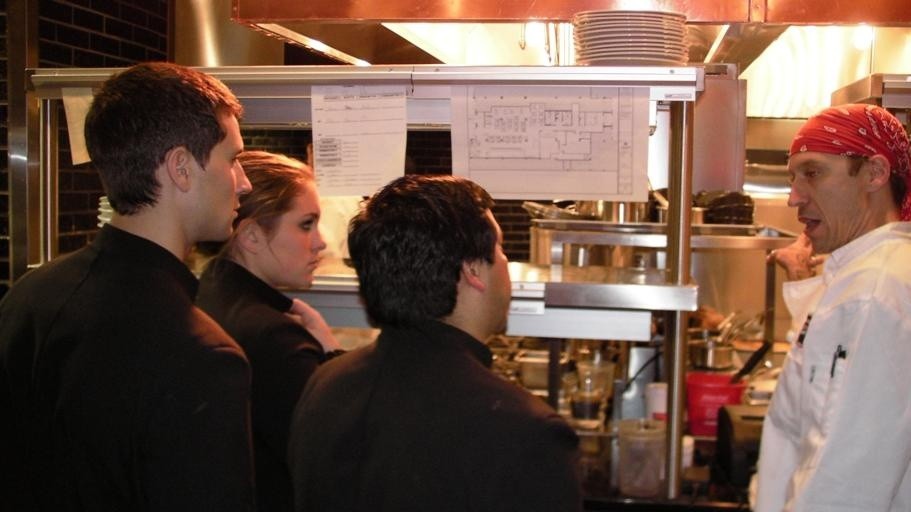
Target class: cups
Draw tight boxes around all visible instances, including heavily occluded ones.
[579,361,616,399]
[572,390,608,427]
[605,418,666,497]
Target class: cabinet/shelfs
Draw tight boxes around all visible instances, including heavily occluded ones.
[24,66,703,510]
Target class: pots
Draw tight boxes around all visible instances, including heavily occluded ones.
[689,308,766,369]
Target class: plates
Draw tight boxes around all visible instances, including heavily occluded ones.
[572,10,690,65]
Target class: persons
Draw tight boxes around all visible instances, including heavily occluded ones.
[3,61,257,512]
[285,172,586,512]
[748,100,911,511]
[190,146,352,510]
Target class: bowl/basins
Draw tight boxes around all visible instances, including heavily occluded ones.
[746,378,780,403]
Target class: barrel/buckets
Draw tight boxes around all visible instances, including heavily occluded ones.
[682,371,749,435]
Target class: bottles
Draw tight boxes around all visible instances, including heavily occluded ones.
[679,434,693,476]
[645,380,668,424]
